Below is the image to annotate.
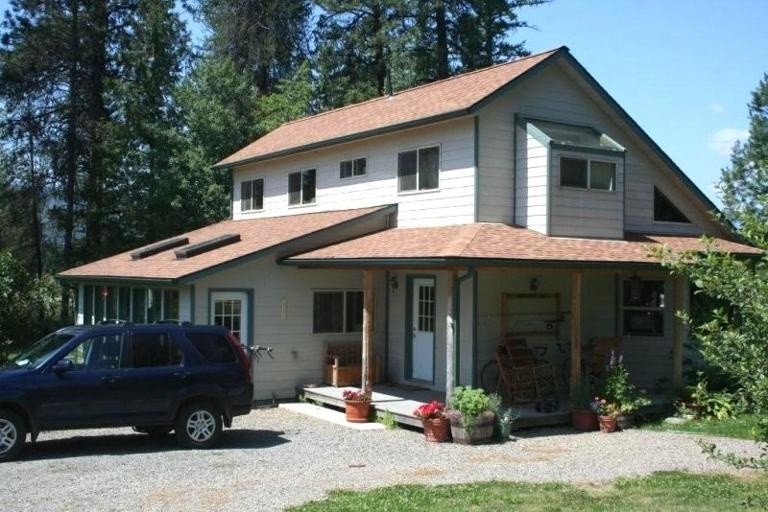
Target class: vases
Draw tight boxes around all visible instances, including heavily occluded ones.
[344,398,372,423]
[420,415,450,442]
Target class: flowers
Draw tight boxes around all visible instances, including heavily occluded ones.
[412,400,446,420]
[342,389,373,400]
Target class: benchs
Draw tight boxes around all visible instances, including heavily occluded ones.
[321,339,381,389]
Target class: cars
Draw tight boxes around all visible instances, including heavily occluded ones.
[0,319,253,461]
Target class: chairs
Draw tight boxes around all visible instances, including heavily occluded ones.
[496,337,564,406]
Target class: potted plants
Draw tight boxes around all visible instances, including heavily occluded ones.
[447,384,521,445]
[569,348,653,432]
